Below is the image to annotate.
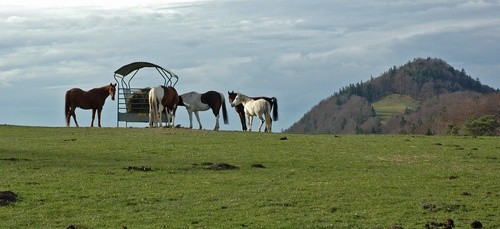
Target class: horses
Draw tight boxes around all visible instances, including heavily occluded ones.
[178,91,229,131]
[227,90,279,133]
[64,82,117,128]
[148,85,178,129]
